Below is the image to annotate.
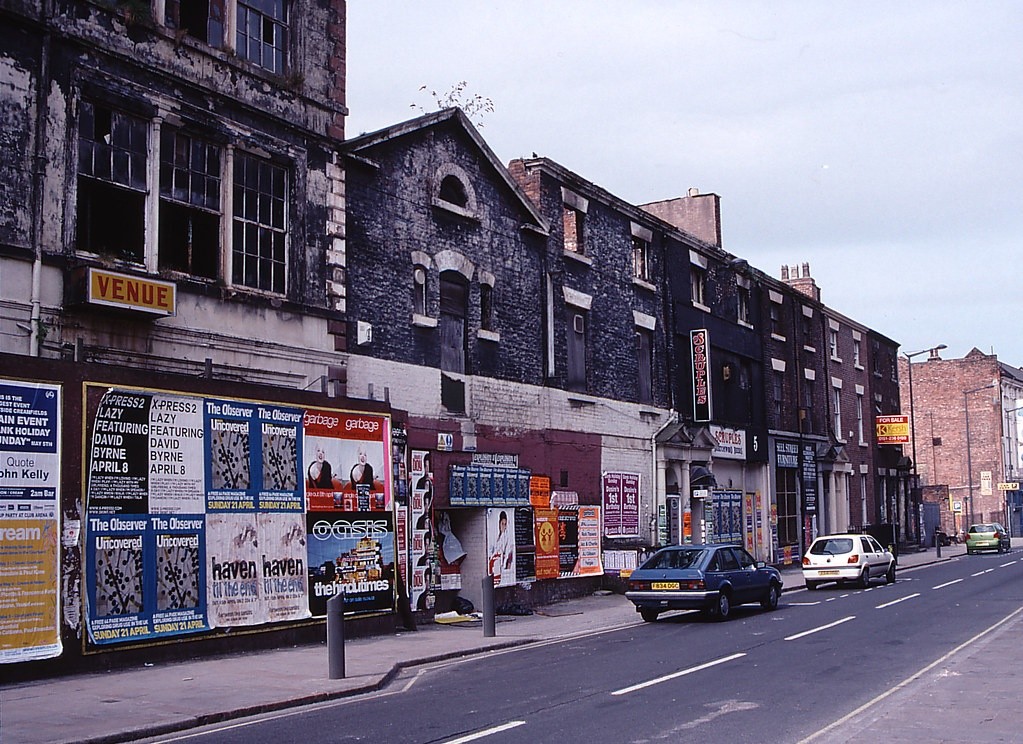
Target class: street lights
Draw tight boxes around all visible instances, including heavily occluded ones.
[962,384,994,524]
[905,343,946,549]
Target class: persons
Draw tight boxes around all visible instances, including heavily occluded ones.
[493,511,513,570]
[417,451,430,611]
[308,443,373,490]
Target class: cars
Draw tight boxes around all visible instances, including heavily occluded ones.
[800,533,894,589]
[966,524,1012,554]
[626,542,786,624]
